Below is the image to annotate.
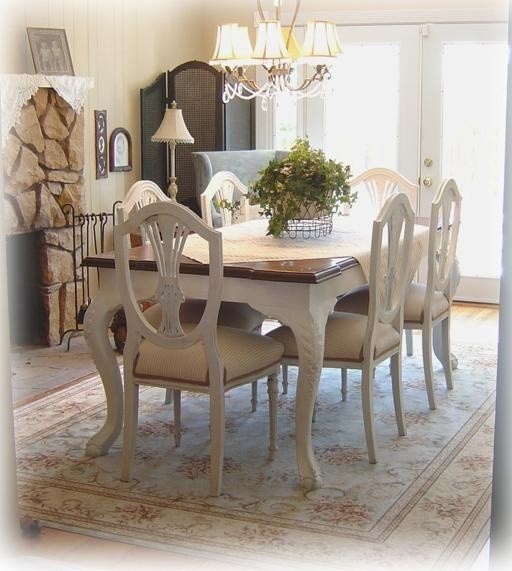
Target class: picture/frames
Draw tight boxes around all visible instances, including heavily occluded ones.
[109,126,133,172]
[27,27,75,77]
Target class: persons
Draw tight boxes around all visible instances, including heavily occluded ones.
[51,41,64,71]
[38,43,54,72]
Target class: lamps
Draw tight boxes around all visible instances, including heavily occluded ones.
[150,99,195,201]
[209,0,344,110]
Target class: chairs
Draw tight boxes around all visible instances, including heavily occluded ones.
[264,192,416,464]
[340,165,420,355]
[200,171,248,230]
[113,201,286,498]
[114,180,266,412]
[334,178,463,411]
[190,149,291,226]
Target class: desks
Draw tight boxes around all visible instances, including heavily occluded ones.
[81,214,458,492]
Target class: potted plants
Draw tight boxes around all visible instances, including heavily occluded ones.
[213,134,359,236]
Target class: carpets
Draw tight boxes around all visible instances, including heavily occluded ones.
[11,319,499,568]
